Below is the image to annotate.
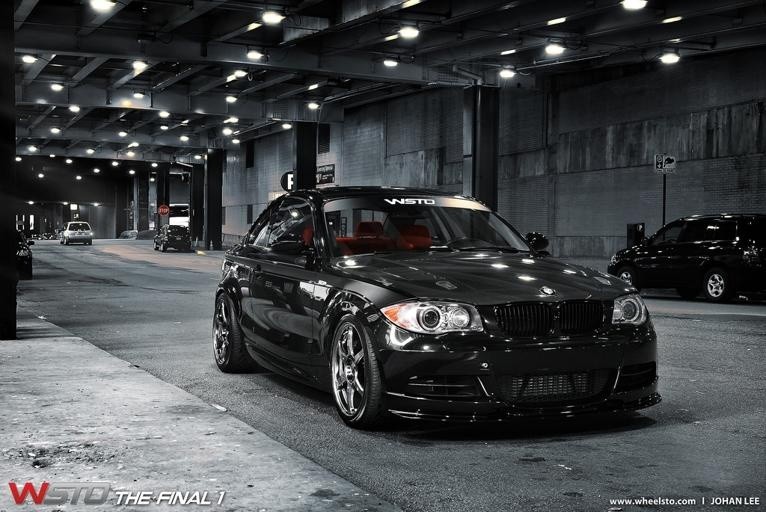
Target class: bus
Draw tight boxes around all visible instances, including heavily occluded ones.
[149,203,190,230]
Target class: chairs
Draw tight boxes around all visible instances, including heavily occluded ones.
[304,228,353,256]
[396,225,434,249]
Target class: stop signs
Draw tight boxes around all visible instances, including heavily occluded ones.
[158,205,170,217]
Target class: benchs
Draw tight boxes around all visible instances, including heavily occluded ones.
[271,222,398,253]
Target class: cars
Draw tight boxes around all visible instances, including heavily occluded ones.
[57,221,93,246]
[15,229,35,280]
[152,224,192,252]
[211,186,663,432]
[607,213,762,304]
